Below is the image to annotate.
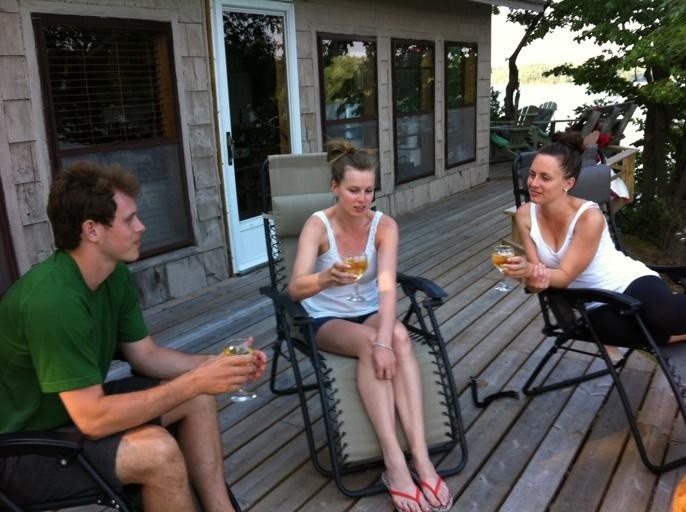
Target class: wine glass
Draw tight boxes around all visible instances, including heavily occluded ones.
[492,243,515,292]
[223,339,259,402]
[344,249,369,303]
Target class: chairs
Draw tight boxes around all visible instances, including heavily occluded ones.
[491,101,557,161]
[259,152,472,500]
[504,144,686,475]
[0,429,243,512]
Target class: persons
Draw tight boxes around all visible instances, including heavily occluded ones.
[501,134,684,347]
[288,139,452,512]
[2,161,267,511]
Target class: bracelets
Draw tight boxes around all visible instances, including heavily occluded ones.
[370,341,396,352]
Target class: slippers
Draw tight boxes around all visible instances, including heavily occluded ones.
[408,461,453,512]
[382,472,433,512]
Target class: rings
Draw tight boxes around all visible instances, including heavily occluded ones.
[541,277,546,283]
